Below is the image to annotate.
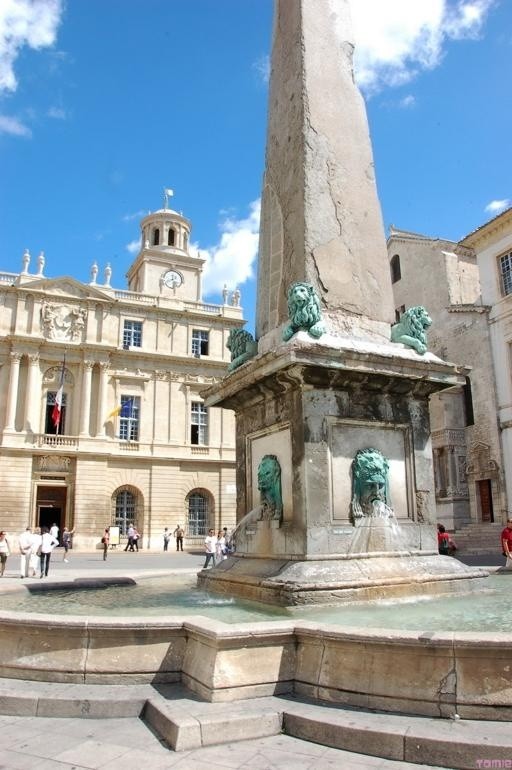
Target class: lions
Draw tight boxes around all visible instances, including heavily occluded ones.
[391,306,433,354]
[281,282,324,342]
[225,327,259,372]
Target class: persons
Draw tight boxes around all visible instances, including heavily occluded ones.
[123,523,135,552]
[500,518,512,571]
[162,527,172,552]
[127,526,140,552]
[62,524,77,564]
[18,521,61,579]
[438,524,450,555]
[0,530,13,578]
[102,528,110,561]
[203,526,231,571]
[174,523,185,551]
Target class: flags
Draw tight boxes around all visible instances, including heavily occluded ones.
[108,397,134,419]
[51,384,63,428]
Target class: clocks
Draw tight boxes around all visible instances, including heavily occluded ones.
[163,269,183,289]
